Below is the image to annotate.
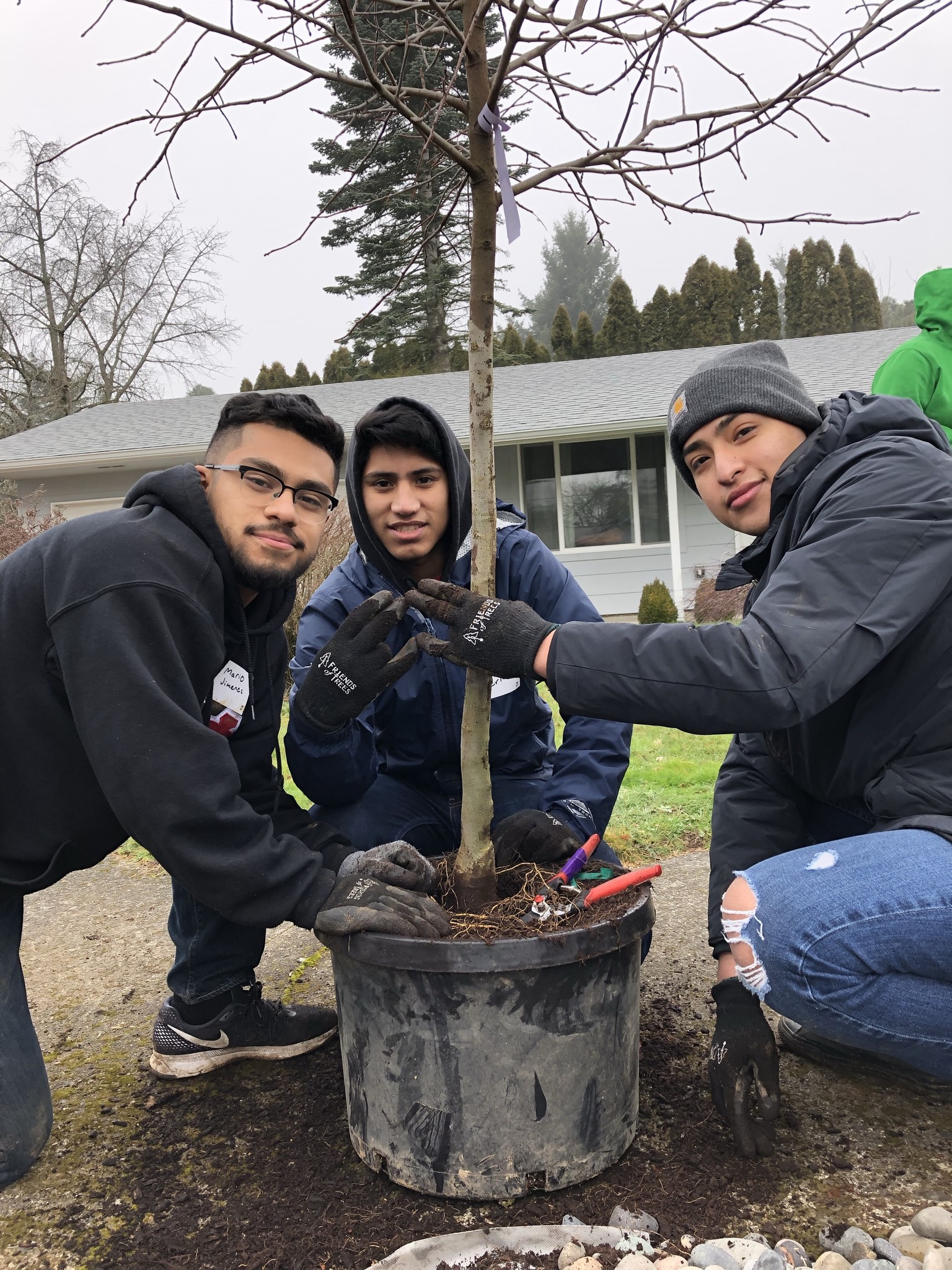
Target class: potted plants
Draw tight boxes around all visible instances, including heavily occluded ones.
[34,0,952,1201]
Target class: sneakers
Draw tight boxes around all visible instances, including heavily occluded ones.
[148,981,338,1080]
[778,1015,952,1098]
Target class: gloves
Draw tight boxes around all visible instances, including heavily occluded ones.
[294,590,419,735]
[404,578,559,681]
[336,840,437,893]
[493,808,582,869]
[314,873,453,941]
[708,976,782,1161]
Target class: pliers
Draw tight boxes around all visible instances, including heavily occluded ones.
[531,832,665,923]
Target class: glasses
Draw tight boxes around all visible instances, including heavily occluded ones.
[203,464,339,525]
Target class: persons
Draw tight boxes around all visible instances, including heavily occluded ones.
[405,345,952,1160]
[872,267,952,447]
[283,395,634,868]
[1,392,453,1190]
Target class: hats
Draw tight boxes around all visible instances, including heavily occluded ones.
[667,338,820,503]
